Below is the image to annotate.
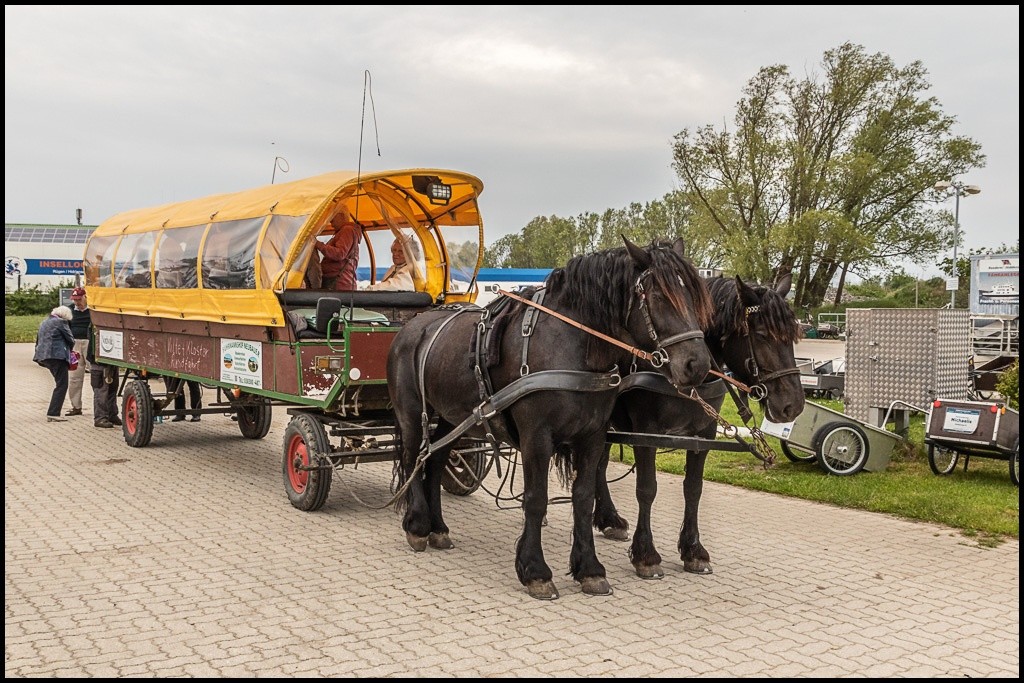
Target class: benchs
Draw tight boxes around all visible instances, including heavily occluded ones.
[281,291,431,322]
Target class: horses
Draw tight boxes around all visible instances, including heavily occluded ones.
[386,235,712,602]
[595,274,805,578]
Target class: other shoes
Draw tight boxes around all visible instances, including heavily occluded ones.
[173,416,186,421]
[65,407,82,417]
[192,417,200,422]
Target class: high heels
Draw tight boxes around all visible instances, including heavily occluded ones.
[47,416,69,422]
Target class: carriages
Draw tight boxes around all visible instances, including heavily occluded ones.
[81,165,807,603]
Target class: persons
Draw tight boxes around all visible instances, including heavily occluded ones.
[32,305,76,422]
[172,376,202,422]
[365,234,421,292]
[86,321,123,428]
[156,204,362,291]
[64,287,91,416]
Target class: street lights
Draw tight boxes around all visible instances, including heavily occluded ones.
[951,185,982,309]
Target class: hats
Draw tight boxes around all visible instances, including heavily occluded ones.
[70,288,86,300]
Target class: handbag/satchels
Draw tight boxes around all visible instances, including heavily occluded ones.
[321,274,337,290]
[66,350,80,371]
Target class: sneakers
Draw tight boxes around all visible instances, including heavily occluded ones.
[110,416,122,425]
[95,418,113,428]
